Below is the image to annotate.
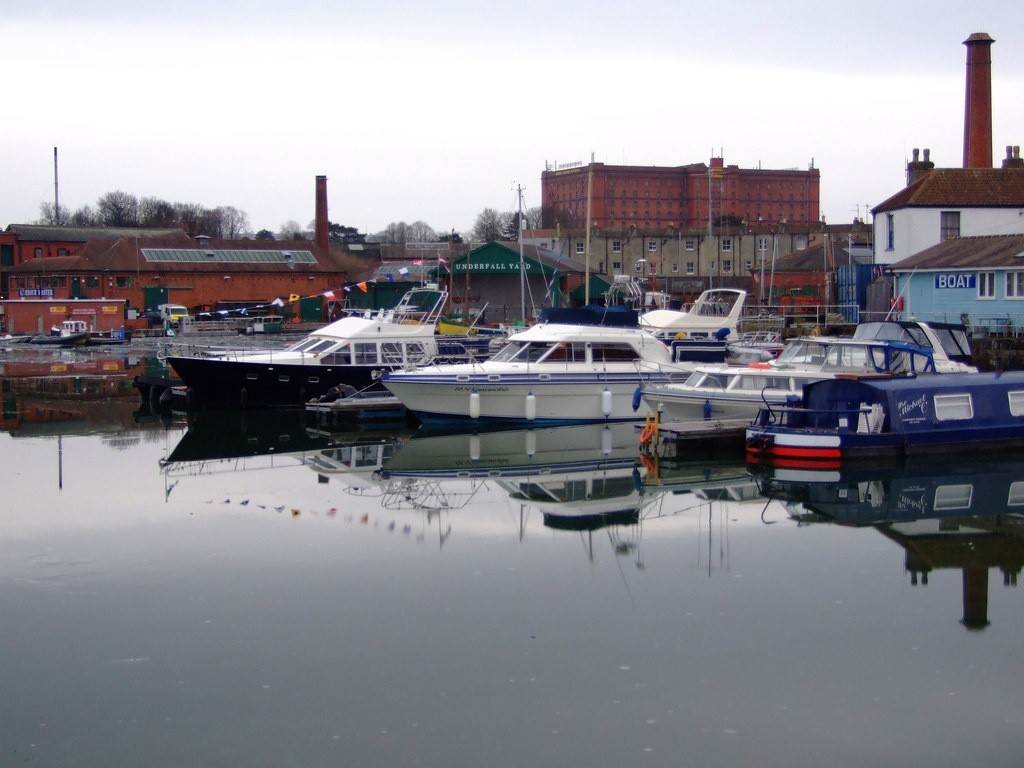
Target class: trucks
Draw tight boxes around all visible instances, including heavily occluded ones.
[161,304,188,328]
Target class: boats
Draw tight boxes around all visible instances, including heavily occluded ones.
[156,421,420,503]
[744,350,1024,483]
[370,173,672,427]
[372,427,666,570]
[0,331,123,348]
[632,465,774,576]
[632,334,936,441]
[758,461,1024,631]
[305,384,408,424]
[156,249,490,407]
[638,288,746,342]
[742,267,979,390]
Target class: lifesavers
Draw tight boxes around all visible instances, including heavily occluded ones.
[641,423,656,444]
[748,362,772,370]
